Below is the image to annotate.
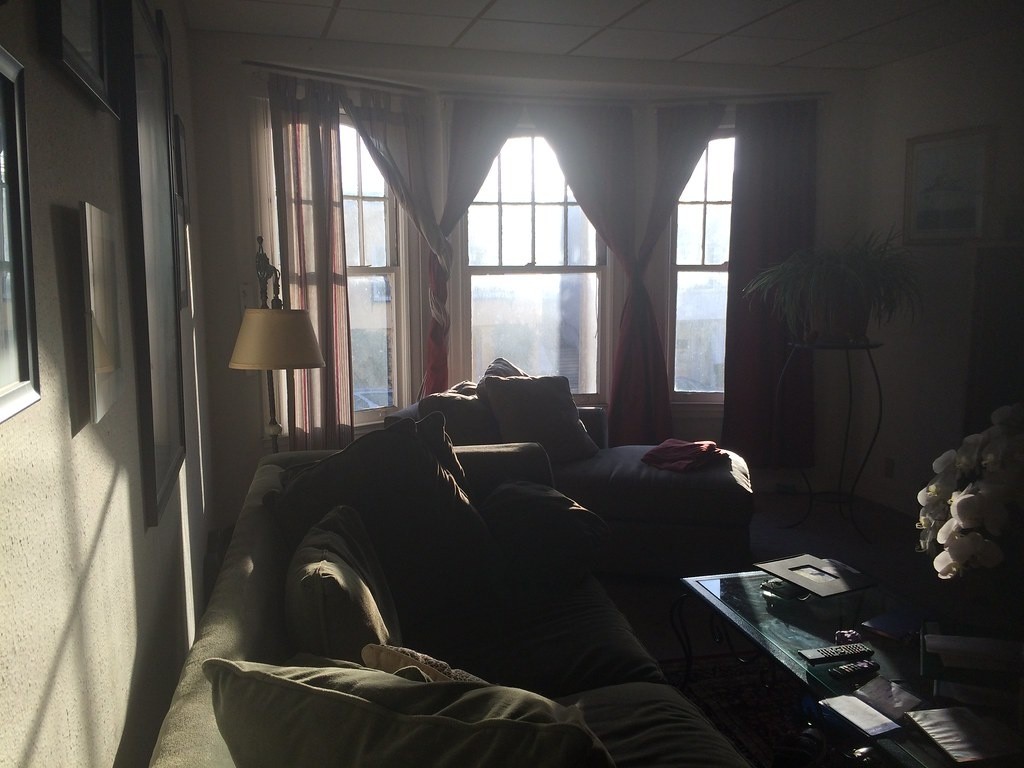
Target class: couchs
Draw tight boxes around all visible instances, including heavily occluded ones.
[384,398,756,587]
[149,441,754,768]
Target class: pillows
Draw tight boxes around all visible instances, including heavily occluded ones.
[262,418,507,602]
[281,503,407,671]
[199,658,615,768]
[484,374,600,462]
[416,358,530,445]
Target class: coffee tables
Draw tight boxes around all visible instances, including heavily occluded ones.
[665,557,1024,768]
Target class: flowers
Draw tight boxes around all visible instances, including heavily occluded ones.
[913,399,1024,601]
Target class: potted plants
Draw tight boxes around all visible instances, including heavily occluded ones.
[742,217,926,344]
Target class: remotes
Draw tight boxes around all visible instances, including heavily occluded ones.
[828,659,880,679]
[797,642,874,667]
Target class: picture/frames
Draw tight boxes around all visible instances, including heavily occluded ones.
[78,198,126,427]
[47,0,124,119]
[0,44,43,424]
[114,2,187,532]
[900,124,998,243]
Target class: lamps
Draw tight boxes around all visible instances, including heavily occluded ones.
[228,235,328,455]
